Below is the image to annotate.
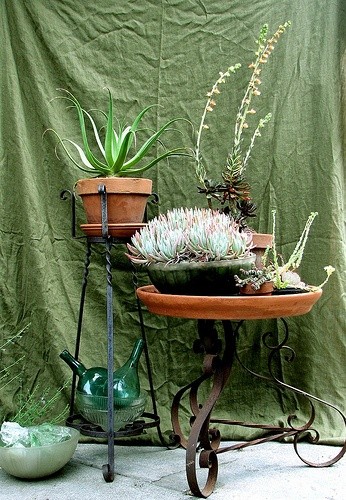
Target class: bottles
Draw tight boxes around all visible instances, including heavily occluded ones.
[59,349,108,412]
[102,338,146,413]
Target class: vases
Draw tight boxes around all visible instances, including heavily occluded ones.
[60,349,109,408]
[102,339,144,408]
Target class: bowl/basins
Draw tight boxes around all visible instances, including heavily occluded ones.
[74,393,148,432]
[0,426,80,480]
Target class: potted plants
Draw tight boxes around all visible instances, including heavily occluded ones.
[234,263,274,296]
[123,206,257,295]
[261,208,335,295]
[192,21,293,279]
[42,87,197,237]
